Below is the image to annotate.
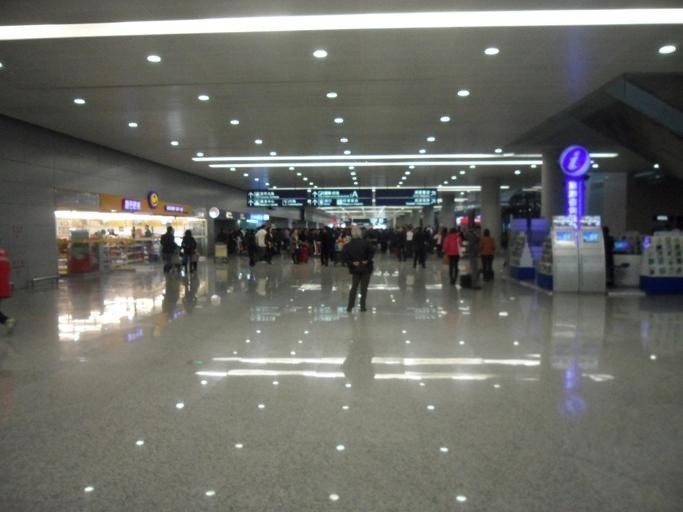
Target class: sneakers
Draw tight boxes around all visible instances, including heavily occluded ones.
[347,304,355,312]
[361,308,368,312]
[4,318,17,335]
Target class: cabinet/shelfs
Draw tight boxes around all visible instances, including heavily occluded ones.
[56,234,158,274]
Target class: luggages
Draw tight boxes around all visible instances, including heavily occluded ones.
[298,244,309,264]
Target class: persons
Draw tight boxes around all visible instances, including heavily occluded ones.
[343,226,374,313]
[602,228,615,286]
[160,227,177,271]
[143,224,151,260]
[180,229,196,272]
[216,224,496,290]
[0,313,16,333]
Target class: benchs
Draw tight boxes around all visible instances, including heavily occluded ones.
[26,273,60,291]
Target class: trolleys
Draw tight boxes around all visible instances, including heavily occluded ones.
[212,242,229,265]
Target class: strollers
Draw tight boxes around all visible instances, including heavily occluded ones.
[170,244,184,272]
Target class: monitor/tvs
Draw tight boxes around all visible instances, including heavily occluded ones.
[556,232,572,240]
[456,215,470,224]
[473,214,481,224]
[582,231,600,243]
